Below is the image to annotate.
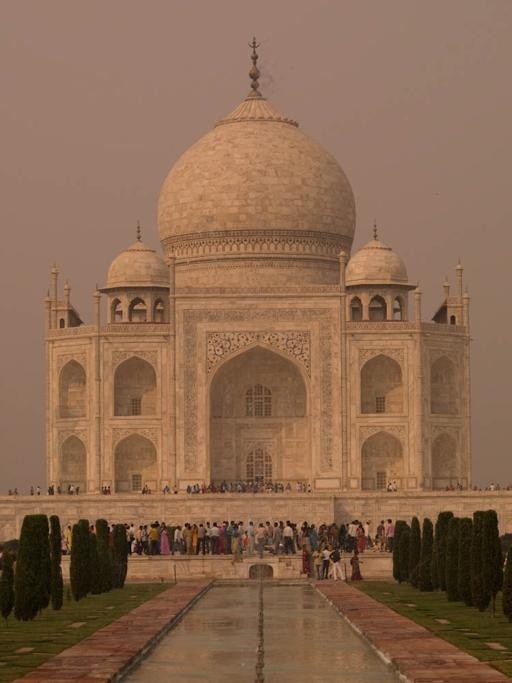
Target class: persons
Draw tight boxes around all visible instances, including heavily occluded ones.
[312,546,326,580]
[386,482,392,491]
[350,549,364,578]
[322,544,335,579]
[229,533,246,565]
[7,479,312,495]
[58,518,395,556]
[446,481,512,490]
[393,480,398,491]
[327,543,347,580]
[302,542,313,576]
